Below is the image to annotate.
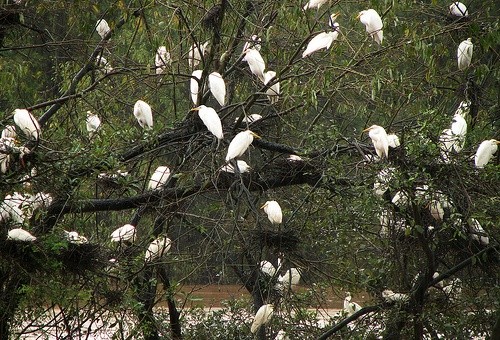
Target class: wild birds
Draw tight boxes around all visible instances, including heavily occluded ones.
[0,190,54,227]
[435,98,500,168]
[298,9,341,59]
[456,37,474,70]
[381,291,409,303]
[258,256,302,288]
[84,110,101,142]
[147,164,171,191]
[342,289,363,320]
[5,226,37,242]
[93,18,113,44]
[153,44,170,75]
[93,54,113,76]
[133,99,154,132]
[288,154,304,162]
[448,1,469,18]
[63,228,88,246]
[108,222,137,244]
[97,167,128,184]
[363,123,403,165]
[0,107,42,189]
[143,234,173,264]
[354,8,385,46]
[299,0,327,12]
[248,301,275,334]
[414,270,463,299]
[187,34,281,177]
[376,167,490,245]
[259,199,284,225]
